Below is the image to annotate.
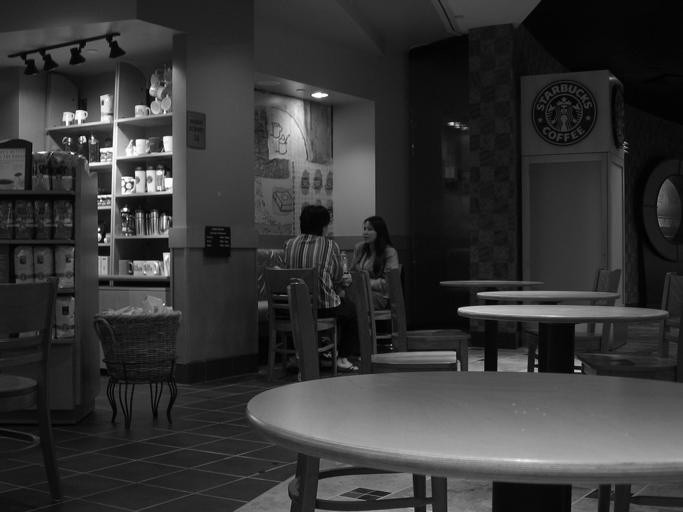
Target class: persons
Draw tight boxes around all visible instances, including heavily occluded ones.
[281,205,359,373]
[322,215,400,360]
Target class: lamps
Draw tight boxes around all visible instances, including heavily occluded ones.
[7,32,126,75]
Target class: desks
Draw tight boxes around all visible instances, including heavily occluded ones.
[250,371,683,509]
[480,289,622,308]
[453,303,673,372]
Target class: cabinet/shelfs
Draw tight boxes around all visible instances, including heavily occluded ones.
[45,63,173,282]
[0,162,100,426]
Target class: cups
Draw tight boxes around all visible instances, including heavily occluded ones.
[133,104,150,118]
[159,93,171,113]
[150,96,162,115]
[0,179,14,190]
[61,111,74,121]
[162,252,170,277]
[120,176,135,194]
[118,258,162,276]
[74,109,88,120]
[135,135,172,154]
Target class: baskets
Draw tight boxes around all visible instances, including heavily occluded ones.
[93,312,182,382]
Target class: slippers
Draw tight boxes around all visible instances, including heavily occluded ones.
[338,364,359,371]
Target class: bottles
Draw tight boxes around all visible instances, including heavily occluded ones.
[62,132,99,163]
[132,207,171,235]
[134,164,172,194]
[340,250,348,275]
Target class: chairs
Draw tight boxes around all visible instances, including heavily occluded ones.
[440,277,542,370]
[352,271,457,375]
[527,267,622,375]
[383,269,471,370]
[579,268,683,377]
[252,270,337,382]
[281,276,447,510]
[0,276,61,499]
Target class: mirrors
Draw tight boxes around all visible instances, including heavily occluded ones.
[641,158,683,263]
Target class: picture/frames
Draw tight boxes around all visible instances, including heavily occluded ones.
[0,140,33,190]
